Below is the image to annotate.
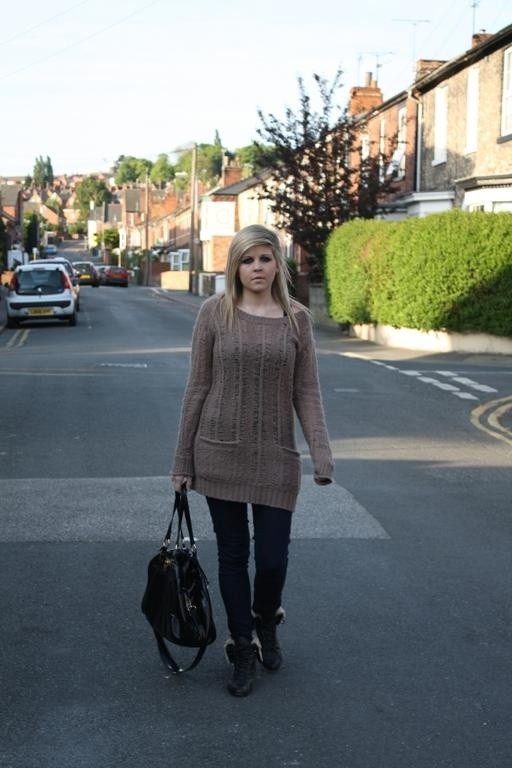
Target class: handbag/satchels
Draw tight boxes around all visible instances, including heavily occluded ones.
[139,483,218,651]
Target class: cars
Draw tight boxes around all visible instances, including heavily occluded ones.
[5,257,128,327]
[47,245,56,254]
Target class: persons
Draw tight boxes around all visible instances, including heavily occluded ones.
[168,225,333,697]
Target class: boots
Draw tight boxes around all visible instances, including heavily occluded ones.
[221,636,264,699]
[249,604,287,670]
